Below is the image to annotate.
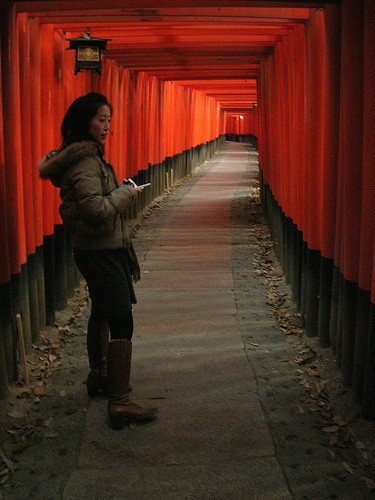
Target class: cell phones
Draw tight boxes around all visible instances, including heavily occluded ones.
[130,183,151,191]
[123,178,138,189]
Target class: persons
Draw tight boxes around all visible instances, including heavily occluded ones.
[37,91,155,428]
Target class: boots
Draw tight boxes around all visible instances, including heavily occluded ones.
[107,338,157,430]
[87,326,133,398]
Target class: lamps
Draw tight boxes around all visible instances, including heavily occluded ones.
[65,27,112,76]
